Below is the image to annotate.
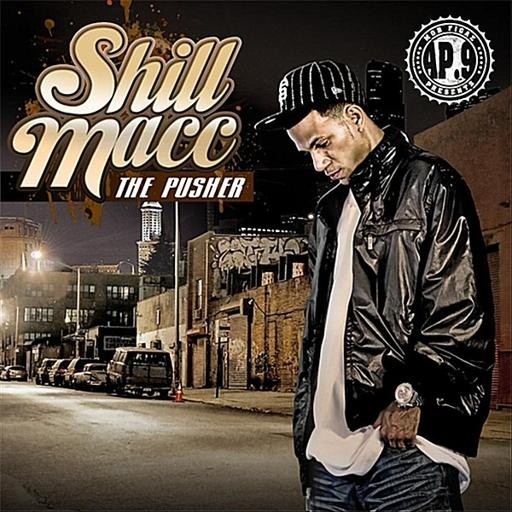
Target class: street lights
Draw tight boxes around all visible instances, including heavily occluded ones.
[30,250,80,359]
[0,303,19,350]
[30,251,81,340]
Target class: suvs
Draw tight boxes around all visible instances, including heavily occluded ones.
[63,357,100,388]
[35,358,57,385]
[48,358,72,386]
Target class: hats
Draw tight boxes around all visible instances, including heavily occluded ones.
[253,58,362,133]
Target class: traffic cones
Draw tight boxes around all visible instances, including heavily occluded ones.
[176,383,184,402]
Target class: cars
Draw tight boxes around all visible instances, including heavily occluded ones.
[1,365,28,381]
[74,363,108,392]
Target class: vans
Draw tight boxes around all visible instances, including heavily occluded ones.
[106,346,173,398]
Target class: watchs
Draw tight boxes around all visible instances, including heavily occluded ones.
[394,381,423,408]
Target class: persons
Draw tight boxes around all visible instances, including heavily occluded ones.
[254,59,497,512]
